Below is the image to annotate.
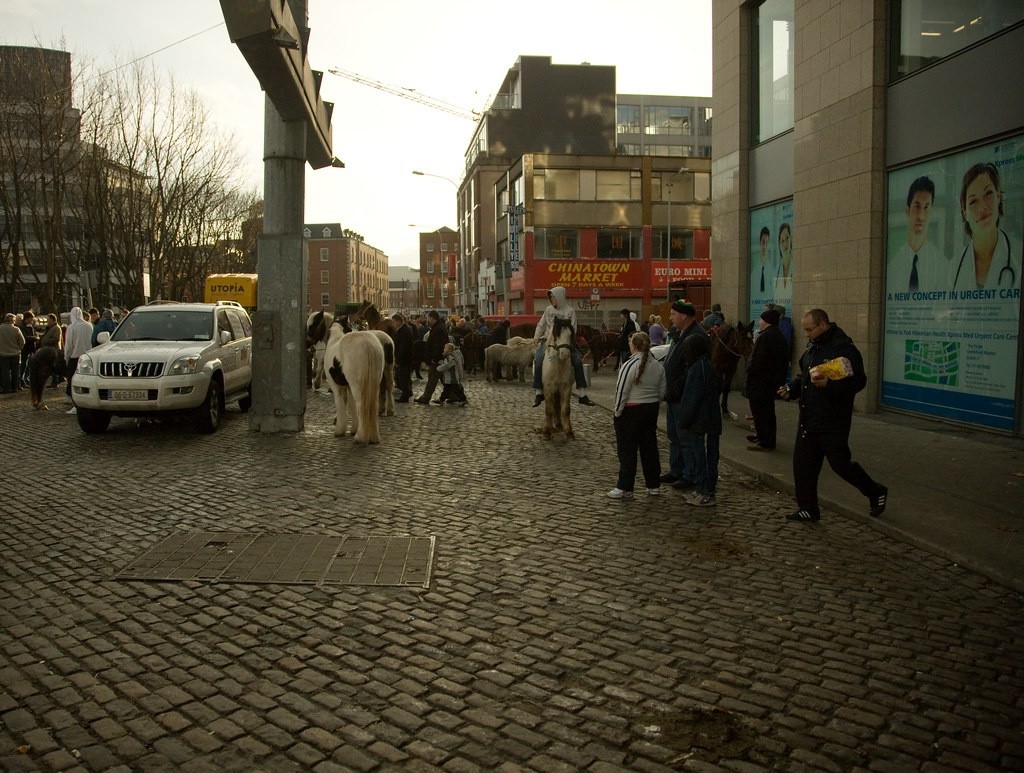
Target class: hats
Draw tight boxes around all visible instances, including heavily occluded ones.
[778,306,786,314]
[761,311,779,326]
[6,315,14,321]
[429,311,439,320]
[711,304,722,311]
[670,299,696,317]
[103,310,114,318]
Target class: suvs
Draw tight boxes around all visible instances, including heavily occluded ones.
[70,299,256,433]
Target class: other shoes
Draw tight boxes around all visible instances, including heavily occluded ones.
[531,393,545,407]
[66,407,77,414]
[607,488,634,498]
[747,445,776,452]
[747,435,759,443]
[18,387,23,390]
[682,491,717,507]
[660,472,699,489]
[578,395,596,406]
[646,486,660,495]
[23,384,30,388]
[46,383,57,388]
[414,399,429,404]
[395,399,408,403]
[57,377,66,384]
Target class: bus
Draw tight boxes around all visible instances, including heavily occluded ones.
[643,279,711,331]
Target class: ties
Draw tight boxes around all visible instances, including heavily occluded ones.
[759,267,765,293]
[907,255,920,292]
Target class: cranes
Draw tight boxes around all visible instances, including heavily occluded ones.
[327,64,492,126]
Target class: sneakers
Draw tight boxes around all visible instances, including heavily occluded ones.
[868,481,888,517]
[785,506,820,521]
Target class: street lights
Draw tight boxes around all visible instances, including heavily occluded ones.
[409,224,444,309]
[412,171,466,317]
[666,167,690,303]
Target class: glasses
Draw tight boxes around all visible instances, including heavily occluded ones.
[802,325,820,332]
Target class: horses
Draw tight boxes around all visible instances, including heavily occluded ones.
[304,302,626,445]
[708,320,755,418]
[28,347,70,411]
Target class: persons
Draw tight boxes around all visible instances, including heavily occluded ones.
[773,223,792,299]
[950,163,1022,291]
[390,309,510,407]
[888,176,948,293]
[607,299,725,506]
[532,286,595,408]
[753,226,773,299]
[0,307,137,415]
[307,345,316,388]
[741,304,792,451]
[778,309,889,522]
